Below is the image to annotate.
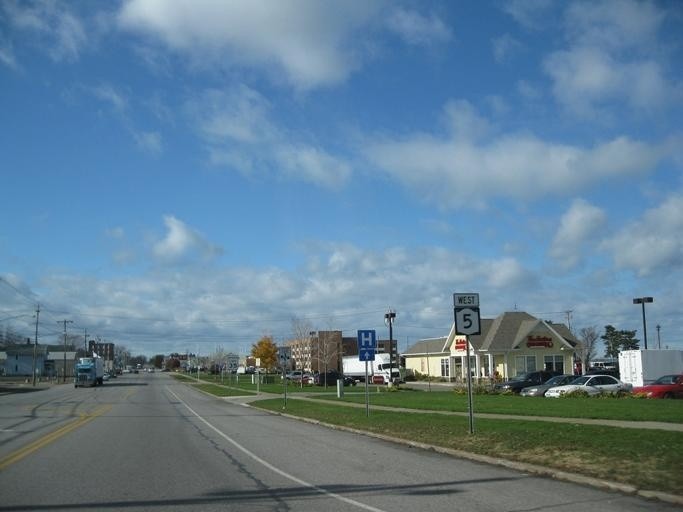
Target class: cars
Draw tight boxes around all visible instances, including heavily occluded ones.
[591,361,616,370]
[103,368,132,381]
[633,375,683,399]
[148,368,155,373]
[494,370,632,398]
[281,371,356,386]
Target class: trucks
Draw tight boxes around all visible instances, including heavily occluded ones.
[343,353,401,385]
[74,358,103,388]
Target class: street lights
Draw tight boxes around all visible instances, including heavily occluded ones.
[633,296,653,349]
[385,313,396,388]
[656,324,662,349]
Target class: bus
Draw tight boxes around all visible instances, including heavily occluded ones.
[136,363,142,369]
